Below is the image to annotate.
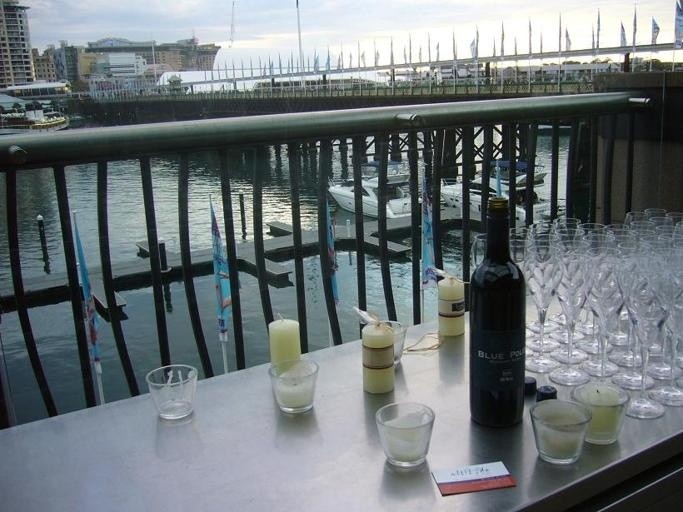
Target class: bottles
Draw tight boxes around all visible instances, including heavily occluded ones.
[468,195,527,430]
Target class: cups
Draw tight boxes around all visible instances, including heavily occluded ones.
[146,364,198,420]
[571,381,630,446]
[268,359,320,415]
[530,399,592,465]
[374,402,436,468]
[381,319,407,365]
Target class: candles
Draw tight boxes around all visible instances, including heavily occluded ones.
[361,321,394,394]
[587,388,618,433]
[438,276,465,336]
[268,313,301,364]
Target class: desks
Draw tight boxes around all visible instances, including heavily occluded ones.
[1,293,683,512]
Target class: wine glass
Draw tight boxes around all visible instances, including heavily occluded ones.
[470,232,487,267]
[508,209,683,421]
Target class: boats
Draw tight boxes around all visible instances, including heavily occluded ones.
[435,177,559,222]
[0,108,71,136]
[325,172,424,222]
[453,163,547,193]
